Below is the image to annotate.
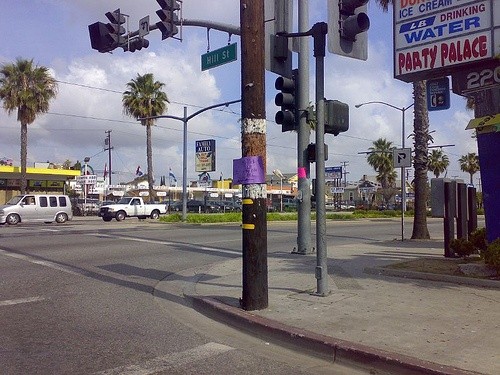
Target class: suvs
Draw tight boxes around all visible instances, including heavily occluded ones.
[82,203,99,215]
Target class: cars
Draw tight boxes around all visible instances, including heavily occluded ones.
[102,201,115,205]
[202,201,220,212]
[169,200,202,212]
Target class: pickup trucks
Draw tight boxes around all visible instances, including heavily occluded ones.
[98,197,166,221]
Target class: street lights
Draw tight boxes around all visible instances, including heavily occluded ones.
[358,145,455,212]
[355,101,414,240]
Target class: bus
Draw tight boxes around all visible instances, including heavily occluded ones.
[77,198,98,213]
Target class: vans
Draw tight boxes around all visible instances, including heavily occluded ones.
[0,195,72,225]
[280,198,297,208]
[223,201,240,210]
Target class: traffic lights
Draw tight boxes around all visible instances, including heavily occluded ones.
[105,12,119,44]
[156,0,180,40]
[338,0,370,42]
[275,69,298,132]
[88,21,112,50]
[119,14,125,44]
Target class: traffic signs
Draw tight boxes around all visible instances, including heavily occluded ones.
[393,148,411,167]
[201,42,237,70]
